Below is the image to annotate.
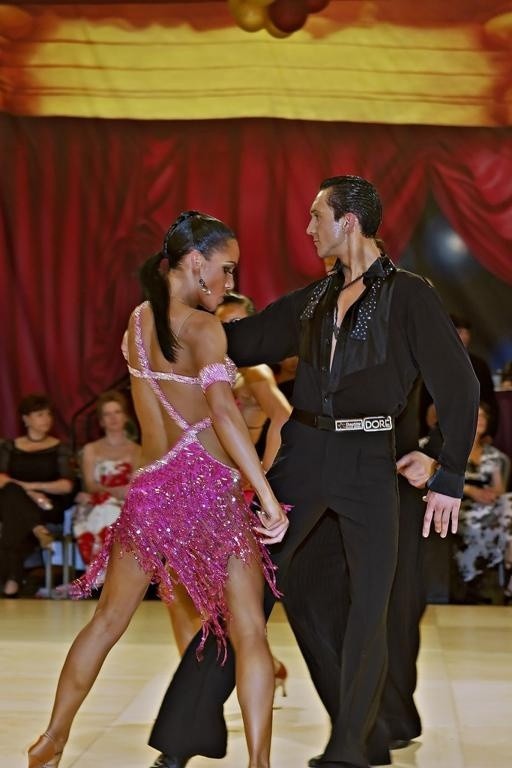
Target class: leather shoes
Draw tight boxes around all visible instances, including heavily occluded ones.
[149,751,191,767]
[290,408,393,433]
[308,740,409,768]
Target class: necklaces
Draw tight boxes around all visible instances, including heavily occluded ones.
[331,268,366,294]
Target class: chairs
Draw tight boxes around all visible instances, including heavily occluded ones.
[42,492,512,594]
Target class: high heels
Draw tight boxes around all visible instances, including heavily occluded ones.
[28,732,62,768]
[275,662,288,697]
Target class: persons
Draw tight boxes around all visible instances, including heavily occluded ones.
[163,281,292,700]
[281,378,445,748]
[24,207,289,768]
[143,172,482,765]
